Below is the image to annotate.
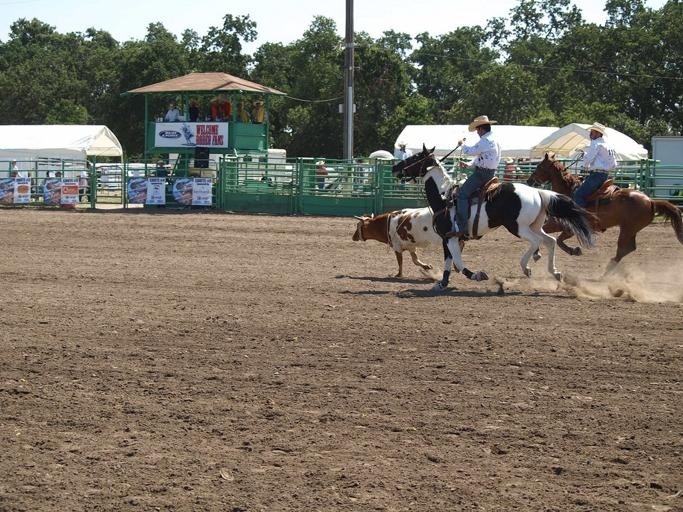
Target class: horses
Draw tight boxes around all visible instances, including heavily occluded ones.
[526,153,683,282]
[391,142,599,292]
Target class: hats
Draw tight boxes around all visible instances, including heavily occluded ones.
[587,122,606,135]
[253,99,264,106]
[316,160,325,165]
[468,115,497,132]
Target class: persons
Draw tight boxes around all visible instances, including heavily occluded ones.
[152,160,170,176]
[195,162,205,168]
[163,92,265,123]
[451,158,469,183]
[578,165,585,183]
[353,159,364,184]
[10,159,22,178]
[76,175,87,201]
[316,160,329,189]
[638,159,646,191]
[40,171,65,201]
[446,114,500,238]
[572,121,617,208]
[28,171,35,189]
[503,158,530,179]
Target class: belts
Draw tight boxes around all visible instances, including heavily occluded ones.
[592,170,608,173]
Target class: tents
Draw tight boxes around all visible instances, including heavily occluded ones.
[0,125,123,178]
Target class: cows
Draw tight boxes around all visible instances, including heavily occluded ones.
[352,206,466,278]
